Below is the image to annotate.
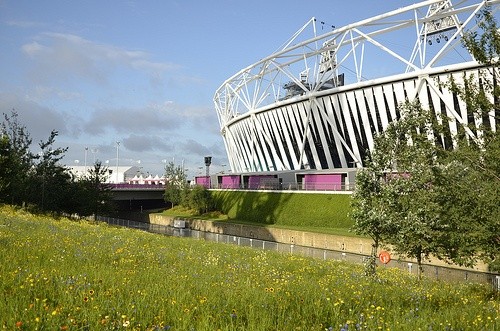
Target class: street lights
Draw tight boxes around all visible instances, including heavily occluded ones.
[116,141,120,183]
[84,146,88,179]
